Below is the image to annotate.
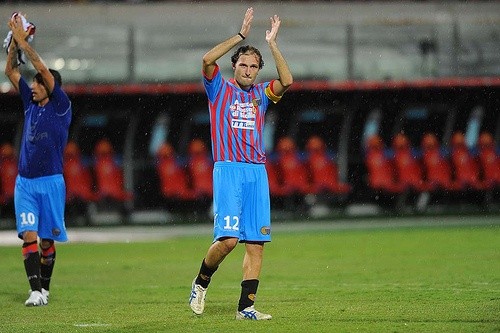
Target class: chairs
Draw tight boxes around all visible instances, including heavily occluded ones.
[159,137,352,222]
[1,139,134,227]
[367,133,500,216]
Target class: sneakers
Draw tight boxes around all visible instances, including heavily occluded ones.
[190,274,208,315]
[236,305,272,321]
[40,288,51,305]
[24,291,45,306]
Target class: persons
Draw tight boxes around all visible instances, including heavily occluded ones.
[189,8,293,321]
[5,12,72,306]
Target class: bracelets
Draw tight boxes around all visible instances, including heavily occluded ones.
[238,33,245,40]
